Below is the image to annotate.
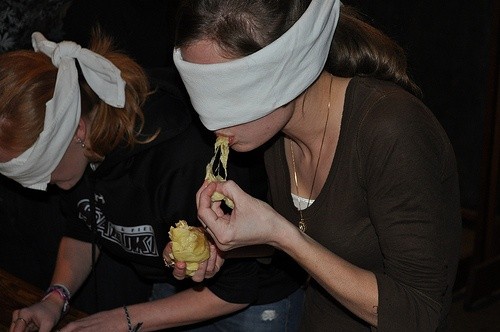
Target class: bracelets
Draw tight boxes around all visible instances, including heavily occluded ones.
[42,284,71,317]
[122,305,143,332]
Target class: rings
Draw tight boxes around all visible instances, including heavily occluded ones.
[16,318,27,327]
[204,225,208,230]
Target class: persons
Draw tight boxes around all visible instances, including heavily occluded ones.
[163,0,462,332]
[0,22,309,332]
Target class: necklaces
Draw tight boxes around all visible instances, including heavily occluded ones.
[289,73,334,233]
